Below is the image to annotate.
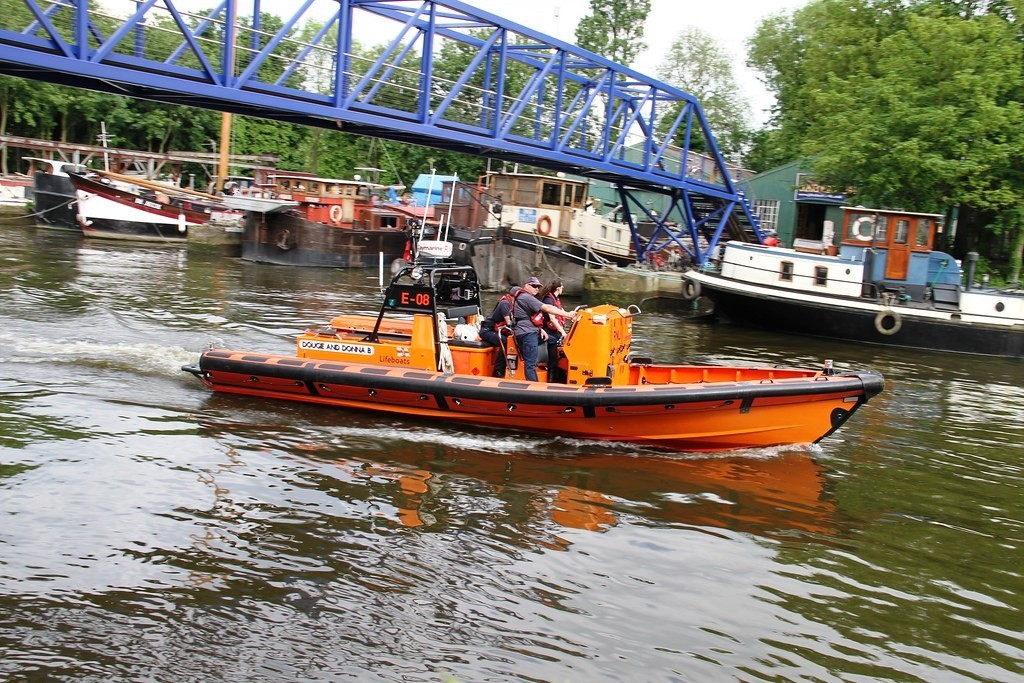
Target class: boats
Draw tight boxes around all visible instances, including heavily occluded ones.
[181,166,891,453]
[1,147,1024,359]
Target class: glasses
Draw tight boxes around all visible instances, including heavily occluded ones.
[528,283,541,290]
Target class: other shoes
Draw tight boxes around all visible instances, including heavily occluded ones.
[549,378,561,383]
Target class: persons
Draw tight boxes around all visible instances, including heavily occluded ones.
[761,237,782,246]
[645,241,727,272]
[584,196,594,211]
[479,276,578,382]
[93,159,385,205]
[648,208,657,222]
[492,190,505,225]
[400,192,410,207]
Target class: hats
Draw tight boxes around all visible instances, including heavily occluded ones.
[524,277,543,287]
[510,286,523,296]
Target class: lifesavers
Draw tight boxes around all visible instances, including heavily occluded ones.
[537,214,551,236]
[875,309,901,334]
[852,216,878,242]
[329,204,343,223]
[681,278,701,300]
